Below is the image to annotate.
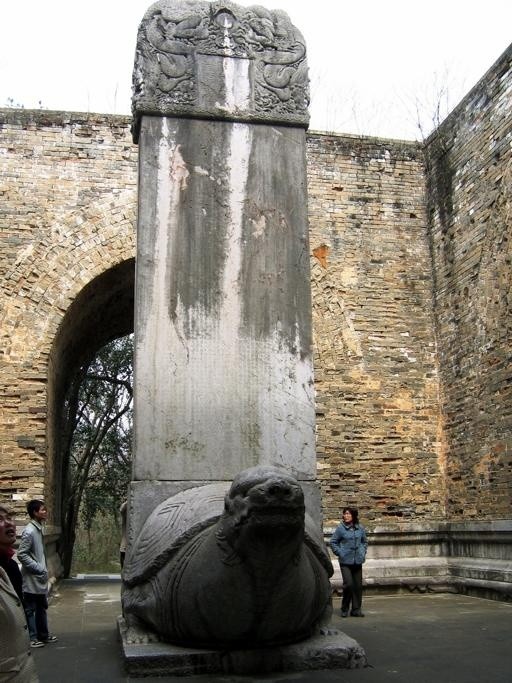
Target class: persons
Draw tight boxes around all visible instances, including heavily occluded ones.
[1,504,44,683]
[118,498,128,566]
[17,498,59,647]
[328,504,367,618]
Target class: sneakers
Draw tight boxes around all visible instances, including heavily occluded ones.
[30,641,45,648]
[341,611,365,618]
[45,637,57,643]
